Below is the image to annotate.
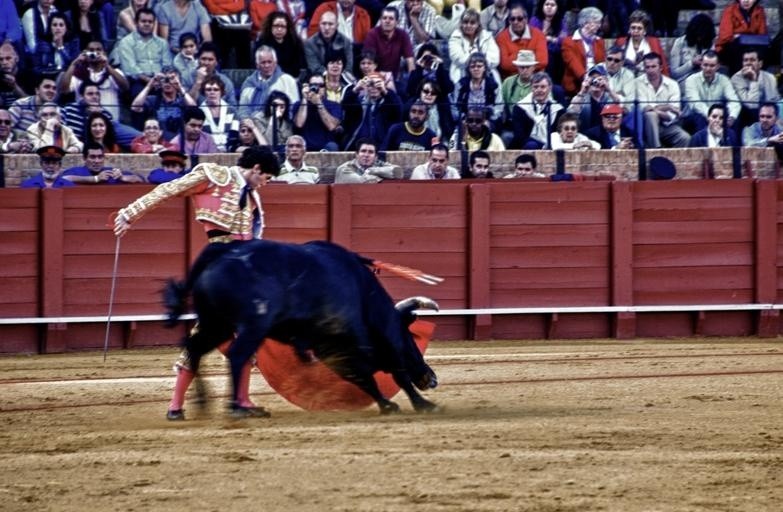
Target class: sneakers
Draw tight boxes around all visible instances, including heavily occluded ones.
[222,399,273,418]
[165,407,185,422]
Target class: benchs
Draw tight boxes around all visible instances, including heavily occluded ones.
[220,68,260,97]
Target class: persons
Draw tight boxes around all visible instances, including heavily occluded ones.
[113,145,280,420]
[1,0,782,189]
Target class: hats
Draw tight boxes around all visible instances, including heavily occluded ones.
[511,49,539,69]
[597,103,625,117]
[34,144,65,162]
[156,150,187,166]
[586,64,609,78]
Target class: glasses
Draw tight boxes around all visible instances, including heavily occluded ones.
[507,15,528,23]
[605,54,624,65]
[563,125,577,132]
[420,62,493,126]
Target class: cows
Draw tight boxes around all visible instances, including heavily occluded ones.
[156,239,440,418]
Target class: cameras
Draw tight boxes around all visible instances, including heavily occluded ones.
[83,50,97,60]
[159,75,170,83]
[422,55,432,61]
[590,77,601,86]
[367,78,381,87]
[308,84,319,94]
